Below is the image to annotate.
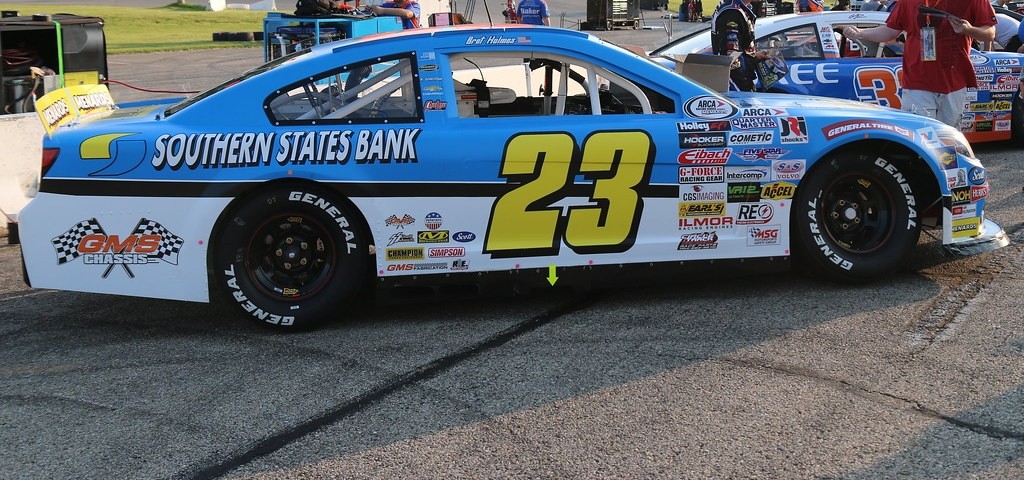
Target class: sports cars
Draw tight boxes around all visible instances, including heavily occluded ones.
[7,20,1013,339]
[645,6,1024,155]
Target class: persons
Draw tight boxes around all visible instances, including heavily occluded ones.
[517,0,550,27]
[838,0,999,130]
[658,0,1024,99]
[365,0,421,30]
